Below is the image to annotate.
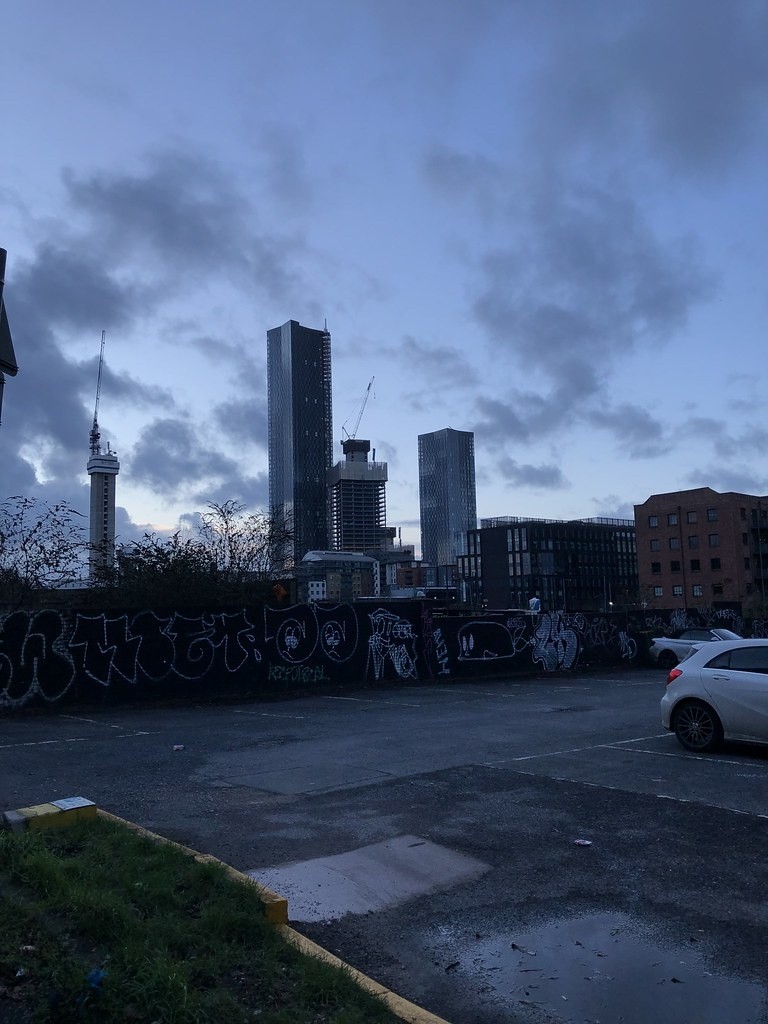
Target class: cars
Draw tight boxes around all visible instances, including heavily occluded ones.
[658,638,768,754]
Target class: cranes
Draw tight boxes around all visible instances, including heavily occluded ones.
[90,330,107,455]
[339,375,376,442]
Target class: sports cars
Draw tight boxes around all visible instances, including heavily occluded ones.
[648,628,745,669]
